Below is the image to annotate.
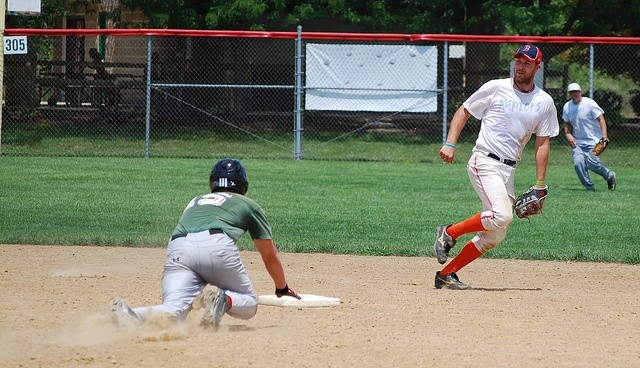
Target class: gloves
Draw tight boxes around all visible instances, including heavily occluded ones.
[275,283,301,299]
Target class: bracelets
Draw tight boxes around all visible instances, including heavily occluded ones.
[443,143,456,148]
[275,284,289,295]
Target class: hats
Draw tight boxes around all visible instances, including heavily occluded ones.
[514,44,543,64]
[568,82,581,92]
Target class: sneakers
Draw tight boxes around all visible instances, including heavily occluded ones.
[433,224,457,264]
[109,297,143,330]
[434,271,470,290]
[608,172,616,190]
[199,285,229,334]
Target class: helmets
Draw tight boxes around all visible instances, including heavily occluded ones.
[209,158,249,195]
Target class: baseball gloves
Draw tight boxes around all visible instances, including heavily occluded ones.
[593,139,609,156]
[513,185,549,218]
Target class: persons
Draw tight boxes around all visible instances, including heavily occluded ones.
[563,83,619,192]
[80,47,113,110]
[434,43,560,288]
[145,52,165,90]
[106,159,302,329]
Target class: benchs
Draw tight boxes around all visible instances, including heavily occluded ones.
[38,61,145,108]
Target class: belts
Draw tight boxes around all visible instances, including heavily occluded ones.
[171,229,223,241]
[488,153,516,166]
